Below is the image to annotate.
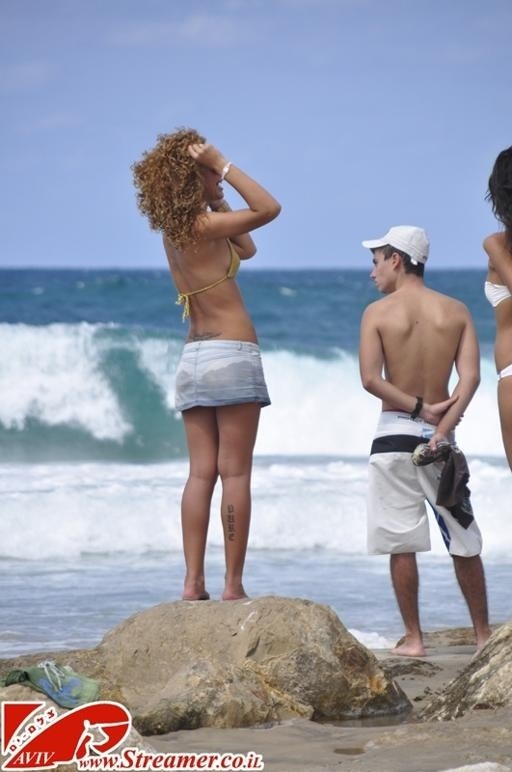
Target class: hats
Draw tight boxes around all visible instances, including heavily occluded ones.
[362,225,427,266]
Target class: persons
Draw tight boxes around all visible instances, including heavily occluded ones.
[129,125,281,601]
[351,225,493,659]
[477,144,509,475]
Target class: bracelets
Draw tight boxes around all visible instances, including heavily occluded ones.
[410,396,423,421]
[221,161,233,180]
